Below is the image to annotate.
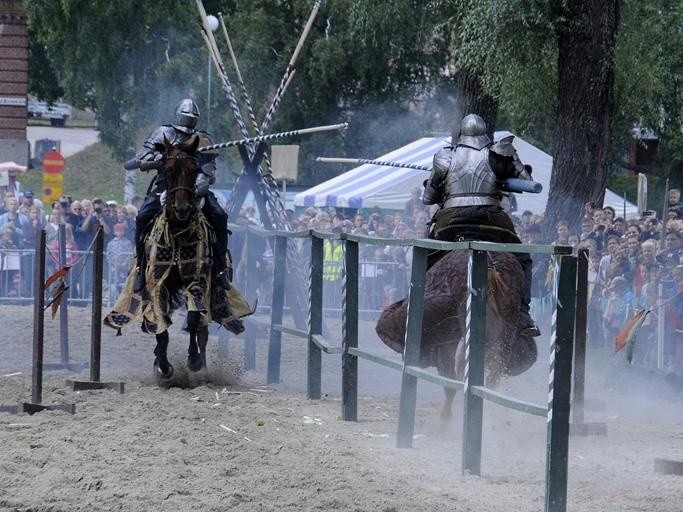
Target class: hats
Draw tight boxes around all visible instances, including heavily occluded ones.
[24,191,33,198]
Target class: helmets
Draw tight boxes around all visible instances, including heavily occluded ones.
[170,99,200,135]
[457,113,492,150]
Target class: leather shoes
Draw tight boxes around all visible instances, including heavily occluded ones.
[214,268,231,290]
[134,266,146,292]
[518,316,541,337]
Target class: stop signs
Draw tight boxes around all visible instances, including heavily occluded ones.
[41,151,65,173]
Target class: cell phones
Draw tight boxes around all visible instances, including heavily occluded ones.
[599,224,606,232]
[643,211,651,217]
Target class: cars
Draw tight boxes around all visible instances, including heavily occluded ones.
[27,94,71,126]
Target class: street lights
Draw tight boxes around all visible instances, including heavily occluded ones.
[204,14,221,138]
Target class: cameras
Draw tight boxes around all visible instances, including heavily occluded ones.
[60,202,67,208]
[95,208,101,214]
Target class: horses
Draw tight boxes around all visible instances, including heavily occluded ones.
[139,132,218,378]
[436,240,526,422]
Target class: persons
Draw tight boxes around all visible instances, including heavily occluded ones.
[0,190,682,379]
[418,113,542,338]
[132,98,230,292]
